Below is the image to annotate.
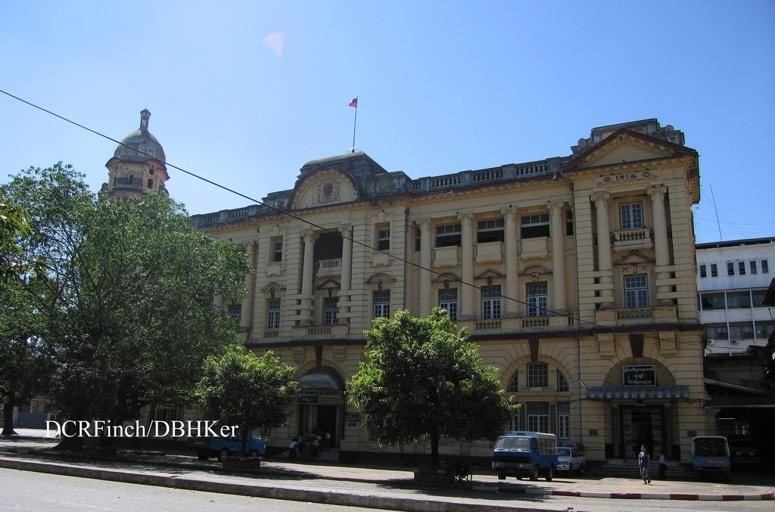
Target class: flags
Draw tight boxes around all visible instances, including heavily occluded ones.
[349,97,357,108]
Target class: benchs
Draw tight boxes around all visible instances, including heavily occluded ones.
[406,452,471,489]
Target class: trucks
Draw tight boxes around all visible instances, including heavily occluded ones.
[192,427,265,460]
[691,435,730,474]
[492,431,558,480]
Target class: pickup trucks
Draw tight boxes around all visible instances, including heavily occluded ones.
[557,446,587,474]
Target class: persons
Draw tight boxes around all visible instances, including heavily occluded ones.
[638,444,651,484]
[288,431,323,462]
